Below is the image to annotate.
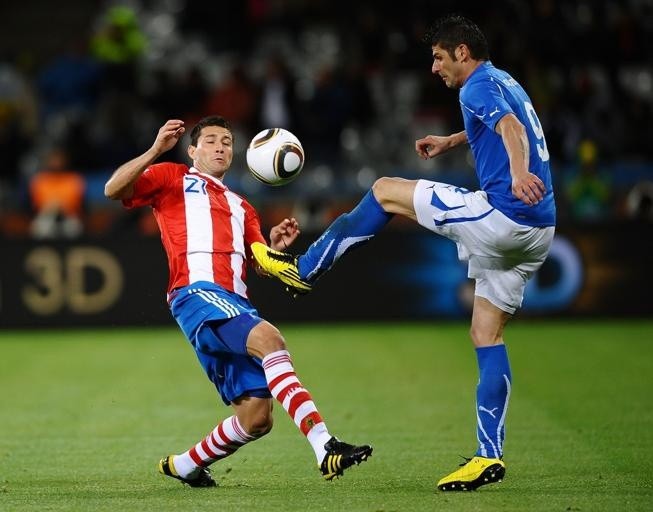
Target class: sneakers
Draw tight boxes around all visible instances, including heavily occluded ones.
[437,454,506,492]
[158,454,218,489]
[317,436,374,481]
[250,241,314,302]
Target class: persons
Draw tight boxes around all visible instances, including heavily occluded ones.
[0,1,653,243]
[103,115,374,486]
[249,15,558,492]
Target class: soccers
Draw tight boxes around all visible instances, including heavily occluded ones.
[246,128,304,187]
